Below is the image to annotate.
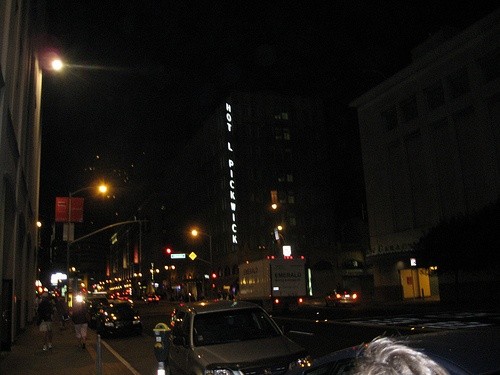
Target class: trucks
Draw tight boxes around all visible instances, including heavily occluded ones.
[235,255,307,311]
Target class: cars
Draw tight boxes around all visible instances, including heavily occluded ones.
[326,287,359,307]
[154,301,314,375]
[146,295,160,304]
[84,297,143,337]
[289,327,500,375]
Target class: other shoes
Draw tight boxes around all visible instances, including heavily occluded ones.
[43,345,47,351]
[82,343,85,349]
[48,343,51,349]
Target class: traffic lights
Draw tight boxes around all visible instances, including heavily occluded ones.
[212,274,217,278]
[166,249,172,254]
[212,282,216,287]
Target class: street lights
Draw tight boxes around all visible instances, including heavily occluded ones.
[191,229,214,300]
[65,185,107,315]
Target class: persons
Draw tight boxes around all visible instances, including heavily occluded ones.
[31,288,89,352]
[349,336,448,375]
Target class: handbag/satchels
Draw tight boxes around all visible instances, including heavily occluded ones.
[40,321,48,332]
